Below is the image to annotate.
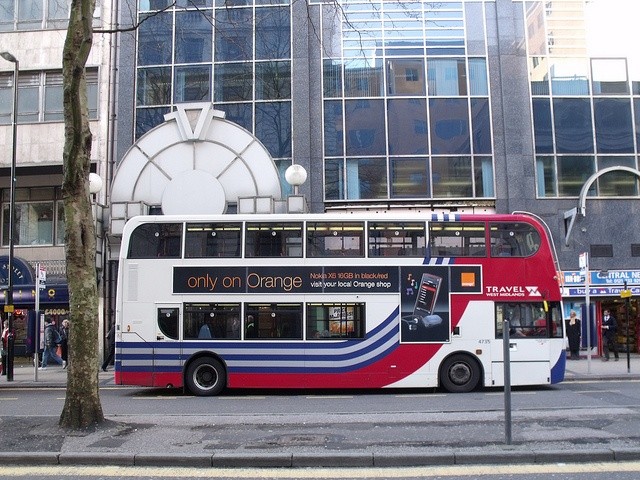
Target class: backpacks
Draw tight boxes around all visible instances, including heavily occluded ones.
[49,326,62,344]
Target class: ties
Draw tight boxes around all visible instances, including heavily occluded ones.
[606,318,608,322]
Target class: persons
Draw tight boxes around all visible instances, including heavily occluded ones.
[101,320,114,372]
[59,319,70,369]
[567,310,582,361]
[0,319,15,376]
[504,311,528,336]
[601,310,620,362]
[38,316,66,369]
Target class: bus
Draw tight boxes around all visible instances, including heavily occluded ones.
[113,212,567,392]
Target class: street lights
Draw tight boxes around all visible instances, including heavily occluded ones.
[0,52,20,382]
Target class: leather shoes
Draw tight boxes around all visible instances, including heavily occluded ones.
[615,357,619,361]
[604,357,609,361]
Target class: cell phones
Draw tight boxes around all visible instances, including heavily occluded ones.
[414,273,442,319]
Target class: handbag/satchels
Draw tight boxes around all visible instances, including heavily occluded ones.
[106,331,111,339]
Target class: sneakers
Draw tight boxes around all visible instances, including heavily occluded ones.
[101,366,108,372]
[62,360,67,369]
[37,367,47,369]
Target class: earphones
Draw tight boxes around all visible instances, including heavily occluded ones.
[409,325,419,332]
[411,319,422,324]
